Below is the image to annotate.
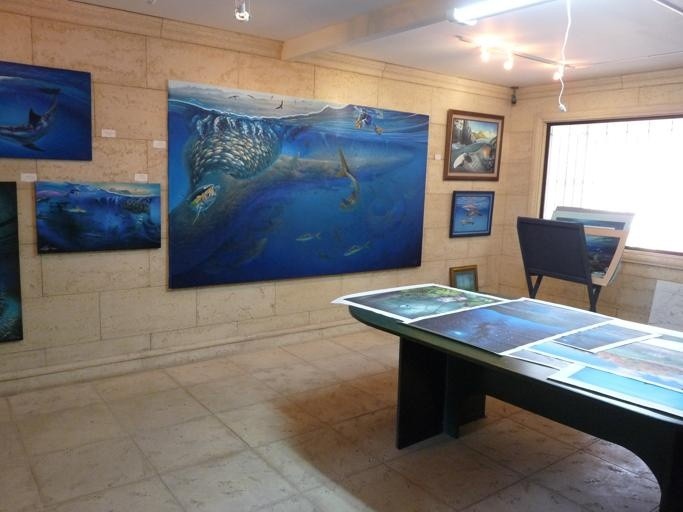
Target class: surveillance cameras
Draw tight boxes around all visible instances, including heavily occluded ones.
[512,95,516,103]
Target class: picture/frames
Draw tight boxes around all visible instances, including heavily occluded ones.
[443,109,505,292]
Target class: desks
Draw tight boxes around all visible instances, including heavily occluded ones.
[347,284,683,511]
